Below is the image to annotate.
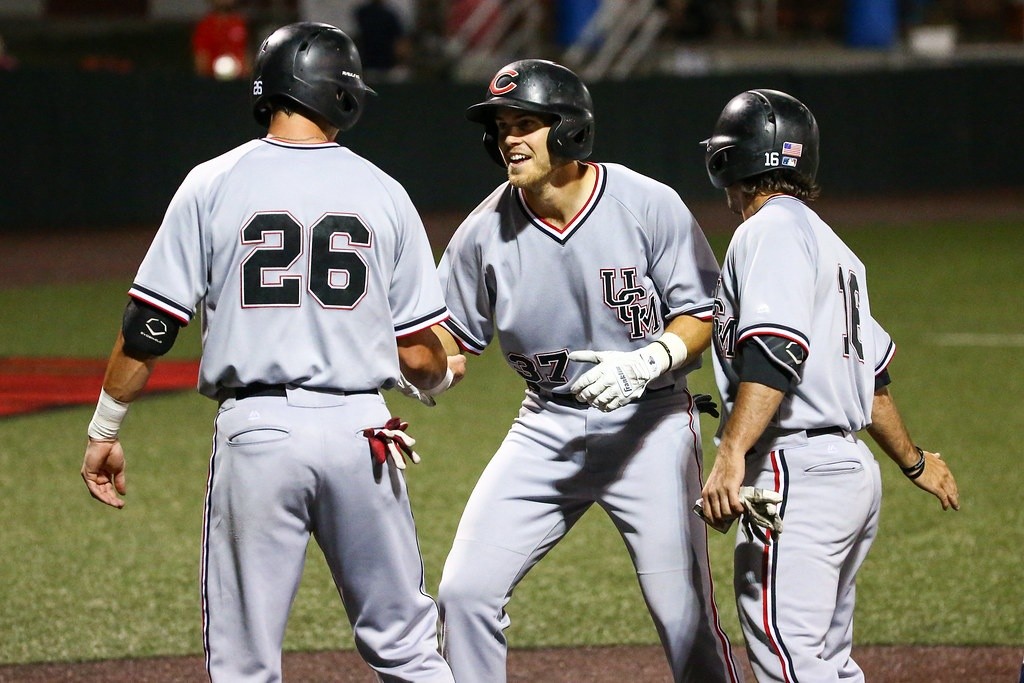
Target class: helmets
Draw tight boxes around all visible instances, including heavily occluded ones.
[252,22,379,131]
[699,89,819,191]
[465,59,594,165]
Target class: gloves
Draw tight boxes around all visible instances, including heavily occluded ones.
[365,415,420,471]
[566,347,663,412]
[692,485,783,546]
[691,391,719,419]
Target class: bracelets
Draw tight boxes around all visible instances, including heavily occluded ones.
[900,445,926,473]
[651,340,672,376]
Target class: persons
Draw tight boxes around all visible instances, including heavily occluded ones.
[79,20,465,683]
[702,89,960,683]
[395,59,746,683]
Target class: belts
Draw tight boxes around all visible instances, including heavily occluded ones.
[218,382,378,400]
[526,381,677,405]
[744,424,843,456]
